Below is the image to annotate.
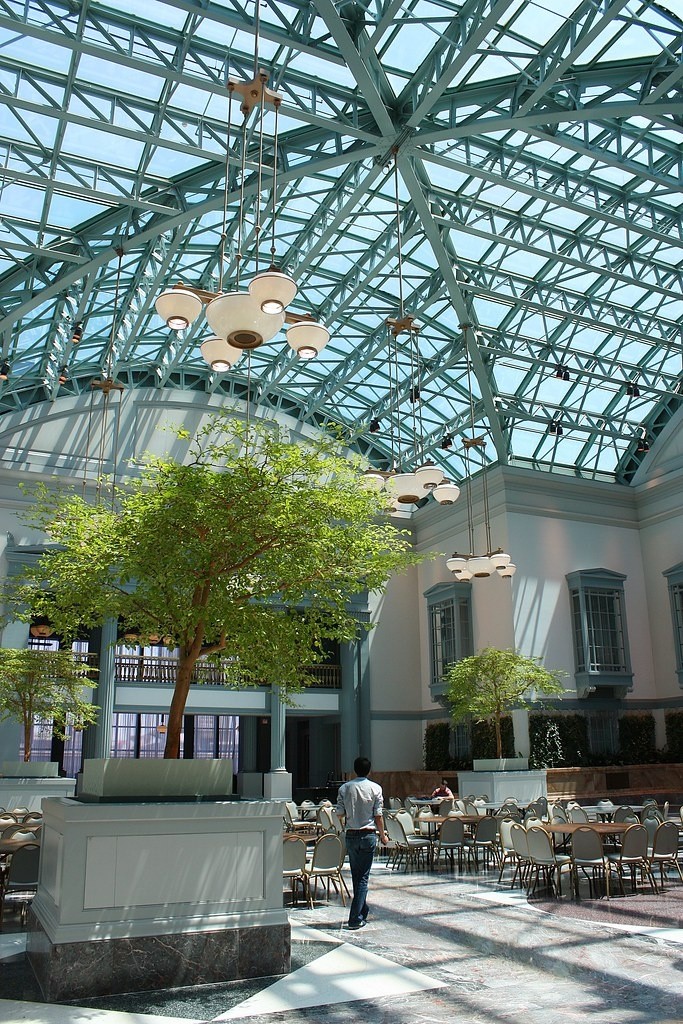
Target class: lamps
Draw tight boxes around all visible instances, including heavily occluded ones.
[155,0,332,372]
[446,323,516,583]
[0,321,84,385]
[30,625,55,640]
[157,713,166,734]
[359,146,460,519]
[370,363,649,453]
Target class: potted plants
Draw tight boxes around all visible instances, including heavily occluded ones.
[0,650,102,813]
[432,648,576,803]
[0,402,445,1002]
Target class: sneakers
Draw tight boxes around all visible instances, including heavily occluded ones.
[349,920,367,929]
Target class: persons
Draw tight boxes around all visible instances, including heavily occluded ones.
[430,780,454,815]
[336,756,389,930]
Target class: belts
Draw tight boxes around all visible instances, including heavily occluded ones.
[347,828,375,833]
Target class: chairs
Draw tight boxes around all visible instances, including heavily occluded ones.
[282,794,683,910]
[0,806,43,929]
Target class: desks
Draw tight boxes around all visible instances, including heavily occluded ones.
[540,823,632,899]
[476,802,531,819]
[282,833,318,841]
[295,805,321,820]
[0,823,40,831]
[579,805,647,823]
[15,813,27,817]
[0,839,40,853]
[411,799,442,804]
[414,815,502,841]
